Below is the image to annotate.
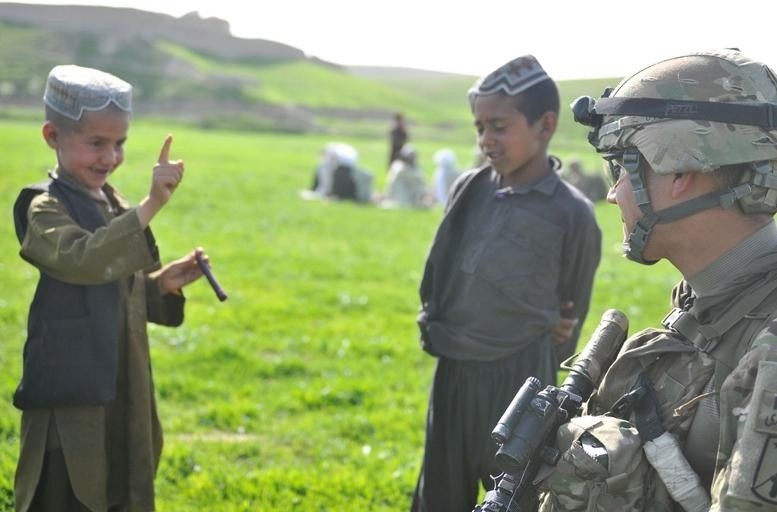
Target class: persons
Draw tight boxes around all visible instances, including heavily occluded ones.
[406,51,604,512]
[386,112,408,166]
[379,141,436,212]
[10,61,226,511]
[426,147,462,206]
[530,42,777,511]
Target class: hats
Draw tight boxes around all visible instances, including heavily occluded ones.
[43,65,132,121]
[468,55,550,116]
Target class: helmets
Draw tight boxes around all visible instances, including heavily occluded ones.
[587,48,775,212]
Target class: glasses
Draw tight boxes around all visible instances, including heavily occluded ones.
[600,152,640,190]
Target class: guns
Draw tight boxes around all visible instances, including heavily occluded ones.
[472,309,628,512]
